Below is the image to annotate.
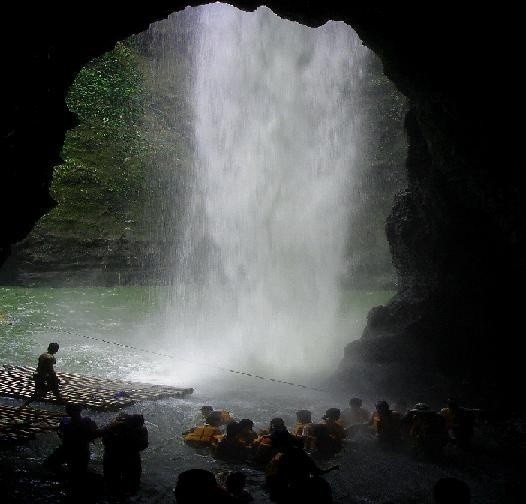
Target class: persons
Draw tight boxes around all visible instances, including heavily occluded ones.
[97,408,150,493]
[44,401,99,475]
[13,341,72,415]
[175,398,500,503]
